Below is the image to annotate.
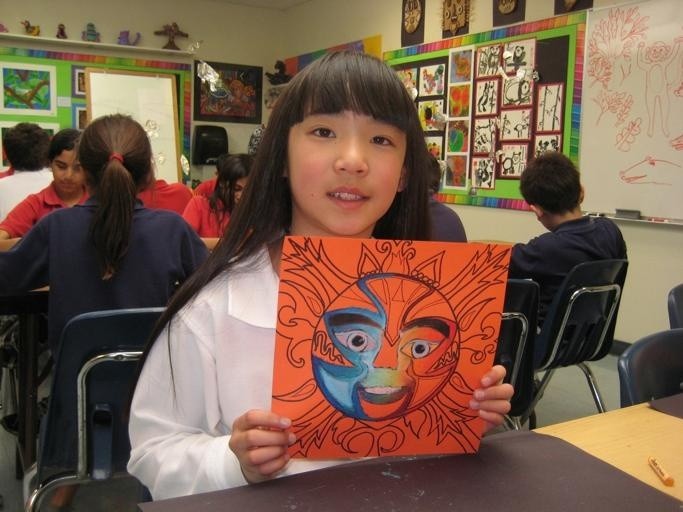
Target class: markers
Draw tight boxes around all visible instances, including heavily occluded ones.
[585,213,604,216]
[648,219,668,222]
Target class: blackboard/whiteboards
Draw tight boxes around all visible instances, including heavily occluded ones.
[578,0,683,225]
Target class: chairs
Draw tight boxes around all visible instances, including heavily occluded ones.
[506,258,629,429]
[30,476,153,512]
[38,305,169,477]
[618,328,683,407]
[499,277,539,432]
[668,284,683,328]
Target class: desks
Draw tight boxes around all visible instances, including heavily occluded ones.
[138,395,681,512]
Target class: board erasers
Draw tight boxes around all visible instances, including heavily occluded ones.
[614,209,640,220]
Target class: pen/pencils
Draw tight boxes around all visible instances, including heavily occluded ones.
[649,456,674,487]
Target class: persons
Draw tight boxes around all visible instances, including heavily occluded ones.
[1,128,92,439]
[192,154,234,202]
[1,113,210,478]
[126,50,516,501]
[501,151,628,416]
[1,122,54,225]
[181,153,258,255]
[425,150,467,242]
[135,179,193,217]
